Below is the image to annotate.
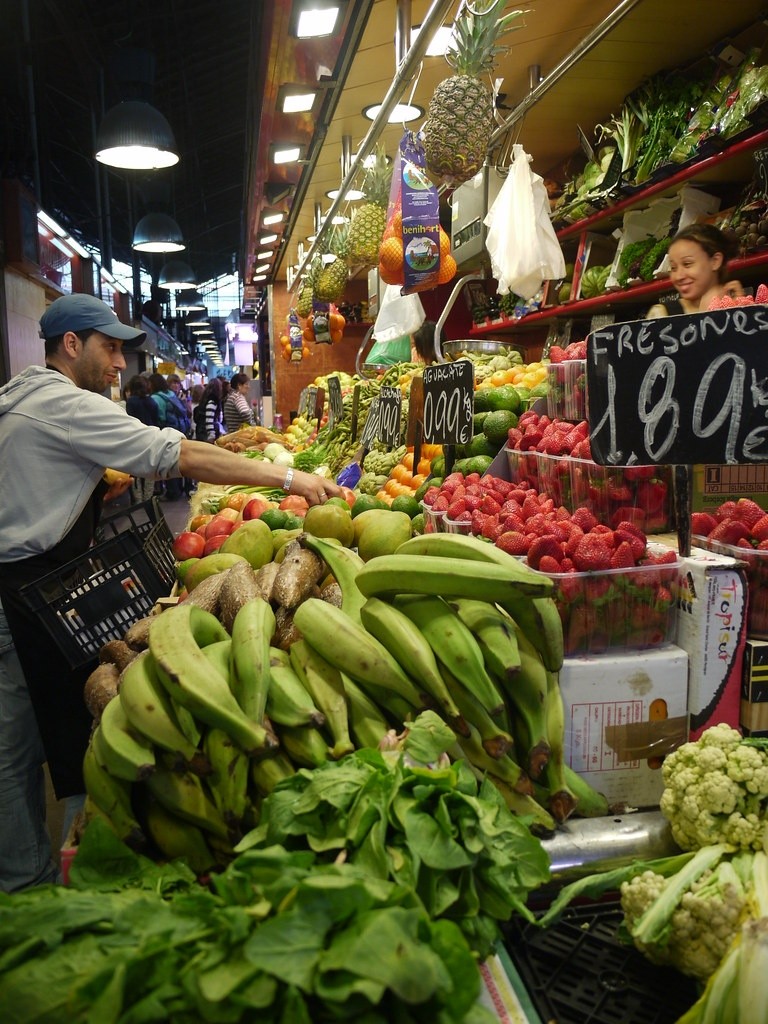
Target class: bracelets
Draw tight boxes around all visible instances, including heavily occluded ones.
[283,468,294,492]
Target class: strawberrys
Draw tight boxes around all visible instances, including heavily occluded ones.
[507,410,670,535]
[689,497,768,635]
[704,282,768,311]
[422,472,678,655]
[547,339,589,420]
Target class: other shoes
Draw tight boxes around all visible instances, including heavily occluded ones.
[159,495,178,502]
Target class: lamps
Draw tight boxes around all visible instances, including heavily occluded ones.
[268,142,305,165]
[130,212,187,254]
[185,311,222,359]
[275,84,323,114]
[260,210,287,226]
[257,232,281,245]
[92,98,182,170]
[288,0,348,40]
[175,289,207,311]
[157,261,200,291]
[263,182,294,207]
[251,248,274,283]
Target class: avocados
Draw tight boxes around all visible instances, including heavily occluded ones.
[258,370,548,539]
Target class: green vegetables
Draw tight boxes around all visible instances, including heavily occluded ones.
[615,235,672,290]
[0,707,550,1024]
[309,359,420,460]
[634,39,768,184]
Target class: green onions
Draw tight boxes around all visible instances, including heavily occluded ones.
[592,80,649,182]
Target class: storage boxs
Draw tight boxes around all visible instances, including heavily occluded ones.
[646,534,750,742]
[504,447,532,484]
[567,455,673,537]
[688,463,768,514]
[603,184,721,292]
[558,642,688,807]
[543,364,565,419]
[560,359,587,422]
[518,550,685,658]
[531,449,572,514]
[541,230,617,309]
[19,493,177,674]
[689,535,768,639]
[738,639,768,738]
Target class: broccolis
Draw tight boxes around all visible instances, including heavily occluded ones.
[618,721,768,988]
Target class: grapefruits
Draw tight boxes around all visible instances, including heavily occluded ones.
[377,210,456,286]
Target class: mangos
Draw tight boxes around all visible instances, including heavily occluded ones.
[174,504,412,603]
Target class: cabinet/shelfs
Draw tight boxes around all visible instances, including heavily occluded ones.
[468,125,768,336]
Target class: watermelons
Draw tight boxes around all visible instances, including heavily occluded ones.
[580,263,614,299]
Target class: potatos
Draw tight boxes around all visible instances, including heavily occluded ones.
[212,425,295,455]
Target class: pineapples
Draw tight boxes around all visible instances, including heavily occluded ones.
[297,141,395,318]
[424,0,535,181]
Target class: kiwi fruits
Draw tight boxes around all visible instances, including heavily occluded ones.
[727,218,768,256]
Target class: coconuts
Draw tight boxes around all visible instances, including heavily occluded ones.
[558,263,575,303]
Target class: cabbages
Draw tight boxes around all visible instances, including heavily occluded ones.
[567,145,616,220]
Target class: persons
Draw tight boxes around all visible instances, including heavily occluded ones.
[0,292,348,802]
[644,223,745,320]
[123,371,255,503]
[413,321,448,365]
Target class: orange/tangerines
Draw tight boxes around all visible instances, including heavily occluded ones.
[280,311,345,362]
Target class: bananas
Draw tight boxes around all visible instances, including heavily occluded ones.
[79,535,611,880]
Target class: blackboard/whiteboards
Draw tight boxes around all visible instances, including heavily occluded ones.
[298,386,318,417]
[361,396,380,450]
[378,386,402,446]
[327,376,343,418]
[422,360,475,443]
[585,302,768,467]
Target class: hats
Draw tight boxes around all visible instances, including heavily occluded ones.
[38,294,147,348]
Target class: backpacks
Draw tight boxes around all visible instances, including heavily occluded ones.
[152,393,191,435]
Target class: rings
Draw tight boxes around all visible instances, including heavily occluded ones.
[319,493,326,497]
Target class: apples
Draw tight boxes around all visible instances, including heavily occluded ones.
[173,484,357,559]
[102,468,130,486]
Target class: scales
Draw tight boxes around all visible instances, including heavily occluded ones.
[354,267,394,381]
[432,161,526,364]
